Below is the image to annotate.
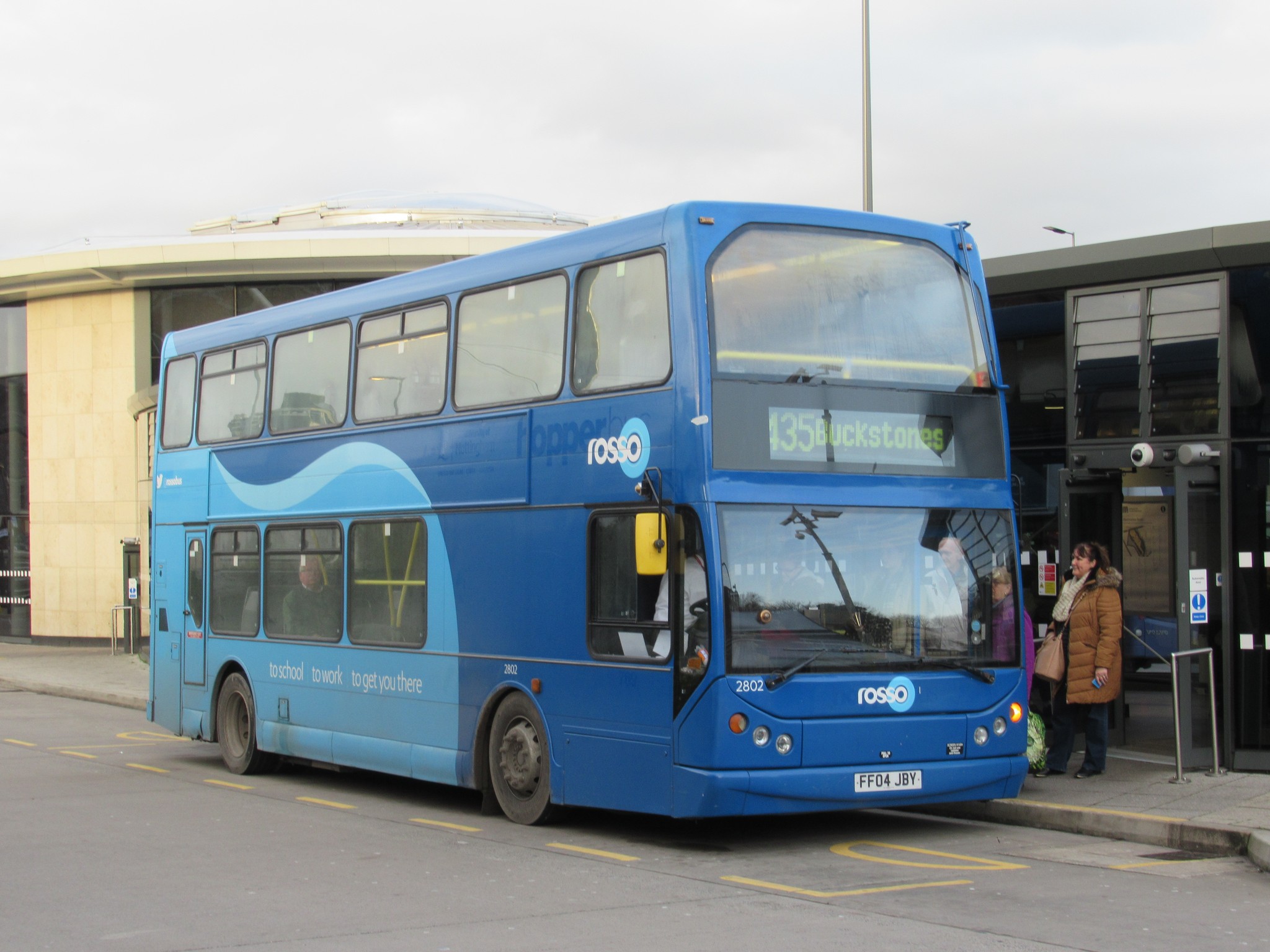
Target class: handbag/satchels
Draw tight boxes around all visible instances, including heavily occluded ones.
[1034,632,1066,682]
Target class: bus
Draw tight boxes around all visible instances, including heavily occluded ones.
[143,199,1029,830]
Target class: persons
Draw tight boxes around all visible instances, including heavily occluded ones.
[282,559,341,637]
[653,549,707,659]
[772,540,824,592]
[1037,539,1123,776]
[864,537,978,658]
[992,566,1035,706]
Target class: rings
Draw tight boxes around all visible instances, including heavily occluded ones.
[1095,676,1106,679]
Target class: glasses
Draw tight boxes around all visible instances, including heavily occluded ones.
[302,568,321,573]
[992,580,1008,585]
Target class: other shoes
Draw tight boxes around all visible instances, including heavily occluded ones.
[1035,768,1062,777]
[1075,767,1102,778]
